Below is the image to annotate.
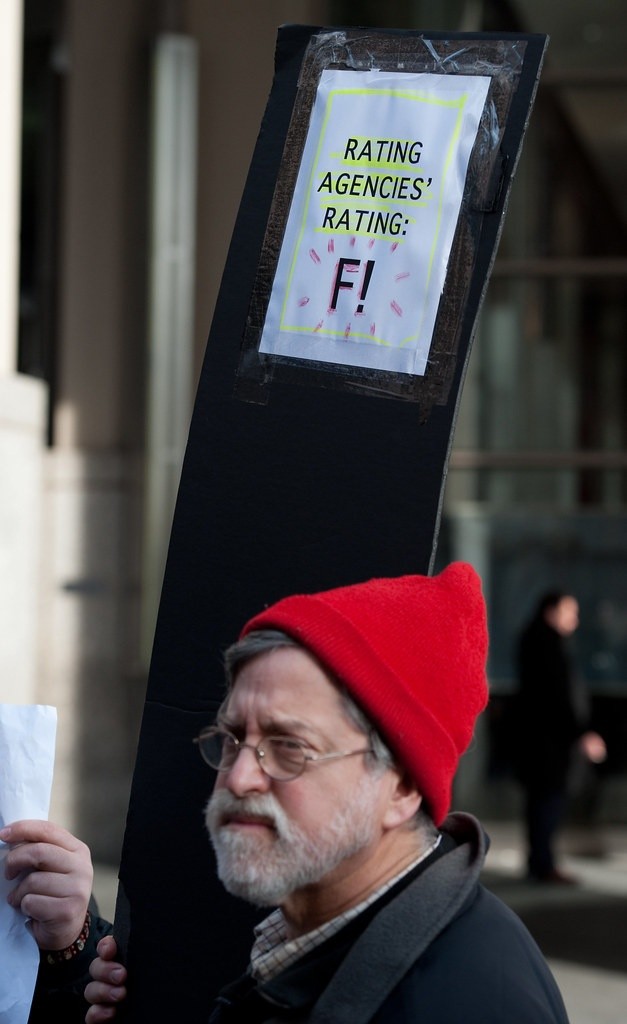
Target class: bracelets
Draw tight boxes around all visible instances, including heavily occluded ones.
[40,912,91,965]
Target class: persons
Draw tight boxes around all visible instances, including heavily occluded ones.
[0,819,114,1024]
[84,562,570,1024]
[514,587,605,888]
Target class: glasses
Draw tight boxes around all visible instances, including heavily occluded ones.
[193,727,374,782]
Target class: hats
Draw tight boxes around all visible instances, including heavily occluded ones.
[238,561,490,827]
